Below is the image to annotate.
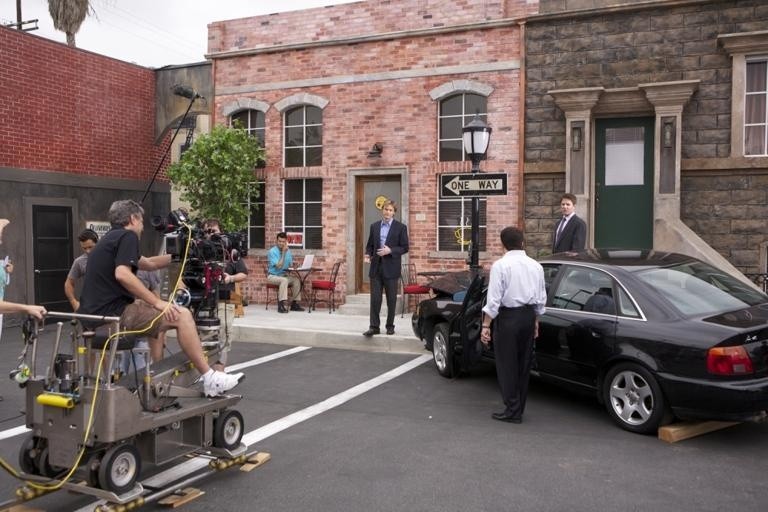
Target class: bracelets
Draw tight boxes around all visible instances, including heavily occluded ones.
[229,274,235,284]
[481,325,490,329]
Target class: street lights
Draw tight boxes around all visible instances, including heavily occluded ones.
[459,107,494,266]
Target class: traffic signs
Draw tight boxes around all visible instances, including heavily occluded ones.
[438,170,510,197]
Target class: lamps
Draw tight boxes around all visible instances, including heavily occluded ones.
[663,122,673,149]
[571,127,583,152]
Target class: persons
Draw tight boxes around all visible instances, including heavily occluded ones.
[0,299,48,325]
[581,285,616,315]
[265,232,305,313]
[479,225,549,424]
[559,319,621,378]
[73,199,246,399]
[549,193,587,253]
[63,228,112,383]
[356,198,411,338]
[135,268,166,366]
[198,218,248,372]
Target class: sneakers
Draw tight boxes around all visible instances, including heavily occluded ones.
[204,370,246,398]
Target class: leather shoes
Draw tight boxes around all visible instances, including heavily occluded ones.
[492,413,522,423]
[363,326,380,335]
[290,304,304,311]
[278,302,288,313]
[387,325,394,334]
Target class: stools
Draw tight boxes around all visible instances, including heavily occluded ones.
[84,332,135,375]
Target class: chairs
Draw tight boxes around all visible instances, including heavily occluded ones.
[308,263,341,314]
[399,263,431,319]
[262,264,289,312]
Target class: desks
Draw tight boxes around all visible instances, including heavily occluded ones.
[283,268,321,314]
[416,271,451,299]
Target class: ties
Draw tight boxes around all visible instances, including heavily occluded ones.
[557,218,566,245]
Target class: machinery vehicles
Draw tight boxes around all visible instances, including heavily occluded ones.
[18,307,244,494]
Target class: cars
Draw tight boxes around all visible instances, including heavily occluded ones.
[410,248,767,438]
